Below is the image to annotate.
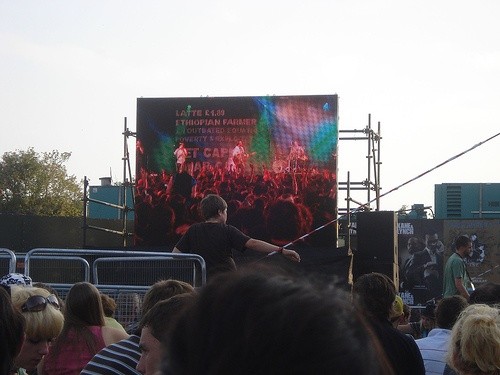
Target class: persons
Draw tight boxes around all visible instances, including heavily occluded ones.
[397,232,485,290]
[0,262,500,375]
[171,194,302,286]
[442,237,474,301]
[136,140,336,247]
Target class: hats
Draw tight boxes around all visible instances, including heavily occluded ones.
[391,296,403,317]
[0,273,32,287]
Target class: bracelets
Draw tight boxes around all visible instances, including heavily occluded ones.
[278,247,283,254]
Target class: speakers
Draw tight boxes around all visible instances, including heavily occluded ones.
[356,211,399,264]
[357,264,400,295]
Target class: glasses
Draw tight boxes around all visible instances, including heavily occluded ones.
[21,294,60,312]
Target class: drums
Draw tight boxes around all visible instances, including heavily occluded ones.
[272,159,287,173]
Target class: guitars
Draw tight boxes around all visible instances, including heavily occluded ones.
[233,151,256,166]
